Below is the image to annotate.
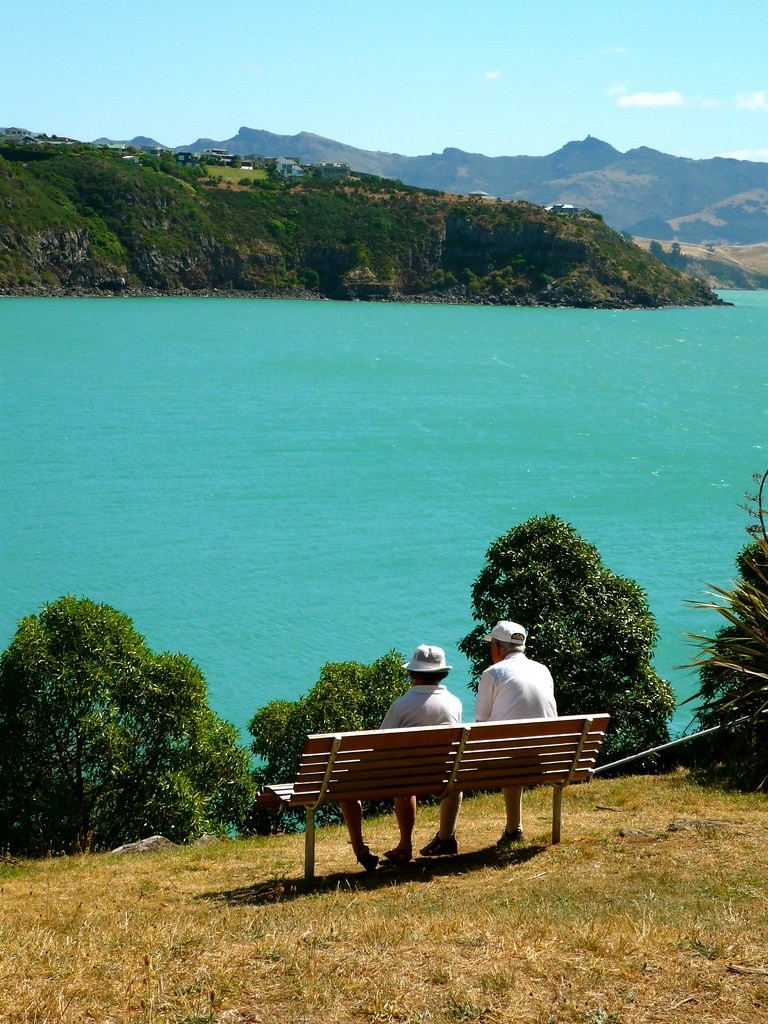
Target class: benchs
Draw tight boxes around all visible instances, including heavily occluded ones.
[253,713,611,881]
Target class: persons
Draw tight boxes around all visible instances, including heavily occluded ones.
[339,644,462,871]
[419,621,558,856]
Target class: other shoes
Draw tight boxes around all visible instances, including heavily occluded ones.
[420,832,458,856]
[497,828,524,847]
[357,845,379,871]
[383,849,413,863]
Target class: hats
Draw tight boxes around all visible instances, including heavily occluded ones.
[483,620,527,645]
[401,644,453,672]
